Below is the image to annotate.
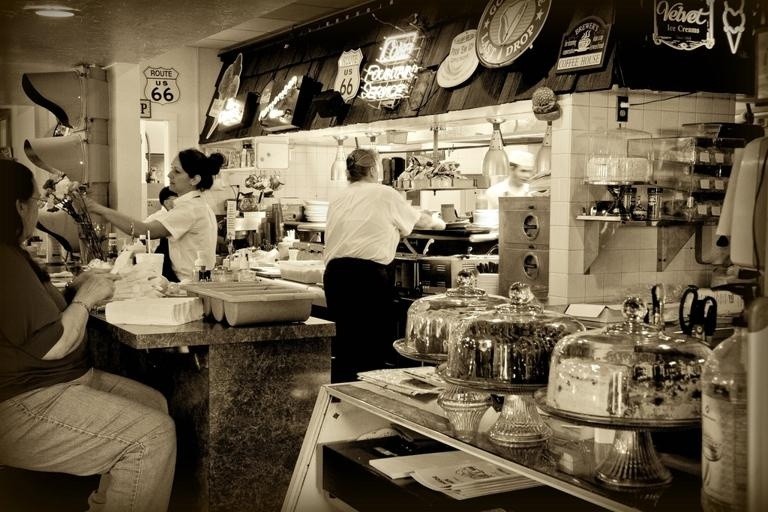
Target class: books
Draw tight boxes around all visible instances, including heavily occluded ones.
[367,448,545,501]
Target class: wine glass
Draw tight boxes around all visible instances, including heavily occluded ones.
[536,116,556,175]
[331,136,349,182]
[365,133,383,184]
[482,116,509,178]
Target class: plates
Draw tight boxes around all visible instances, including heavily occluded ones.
[472,209,500,228]
[304,200,332,224]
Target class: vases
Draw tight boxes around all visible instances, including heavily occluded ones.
[74,221,106,265]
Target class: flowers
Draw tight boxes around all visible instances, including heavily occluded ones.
[43,174,105,262]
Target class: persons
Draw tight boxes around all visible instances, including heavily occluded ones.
[142,185,181,249]
[319,147,447,386]
[72,148,223,284]
[0,161,179,511]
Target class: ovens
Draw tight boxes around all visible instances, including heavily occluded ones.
[501,202,561,298]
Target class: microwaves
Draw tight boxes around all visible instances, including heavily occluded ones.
[390,256,457,291]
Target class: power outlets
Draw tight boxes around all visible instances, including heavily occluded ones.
[618,96,628,123]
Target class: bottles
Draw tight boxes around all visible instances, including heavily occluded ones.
[695,314,748,512]
[104,231,118,262]
[192,253,256,284]
[139,235,147,244]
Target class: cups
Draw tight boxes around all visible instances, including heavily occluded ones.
[225,199,237,253]
[134,251,165,297]
[287,248,301,261]
[441,203,455,224]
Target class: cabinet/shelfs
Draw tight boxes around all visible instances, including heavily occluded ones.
[283,367,702,511]
[204,136,289,173]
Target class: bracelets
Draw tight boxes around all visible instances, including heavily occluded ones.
[69,297,90,315]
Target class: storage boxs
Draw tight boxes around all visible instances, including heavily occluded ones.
[564,304,626,323]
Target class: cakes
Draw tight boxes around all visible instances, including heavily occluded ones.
[413,306,498,359]
[551,346,708,421]
[457,320,571,387]
